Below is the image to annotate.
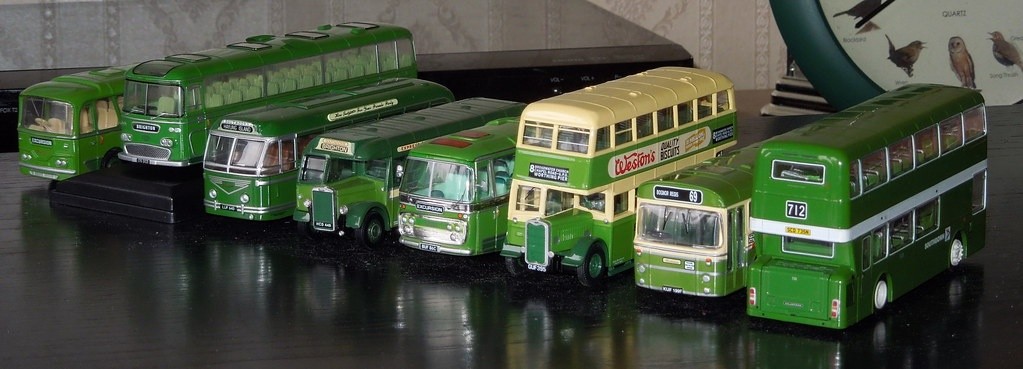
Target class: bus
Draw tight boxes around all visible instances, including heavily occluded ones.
[202,78,456,229]
[632,135,776,298]
[498,66,737,291]
[115,21,418,173]
[292,98,528,248]
[745,83,990,329]
[18,61,140,185]
[392,113,526,257]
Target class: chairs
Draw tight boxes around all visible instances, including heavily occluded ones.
[806,127,978,196]
[874,224,924,258]
[428,190,445,198]
[29,95,124,140]
[156,50,413,113]
[478,154,516,197]
